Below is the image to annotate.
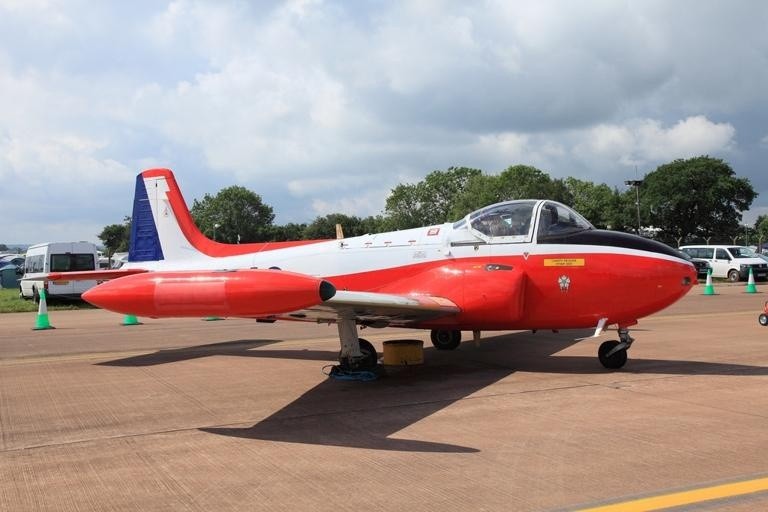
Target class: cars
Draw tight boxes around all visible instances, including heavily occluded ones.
[674,244,768,281]
[0,239,129,299]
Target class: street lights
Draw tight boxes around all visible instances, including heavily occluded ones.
[744,223,747,247]
[212,223,222,240]
[671,233,763,255]
[624,166,646,237]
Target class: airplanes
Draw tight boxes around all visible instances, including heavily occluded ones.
[14,165,703,383]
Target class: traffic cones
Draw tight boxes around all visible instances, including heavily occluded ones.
[31,287,58,333]
[119,312,147,328]
[700,267,720,296]
[201,315,227,324]
[742,265,764,294]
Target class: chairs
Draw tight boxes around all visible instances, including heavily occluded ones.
[489,212,525,237]
